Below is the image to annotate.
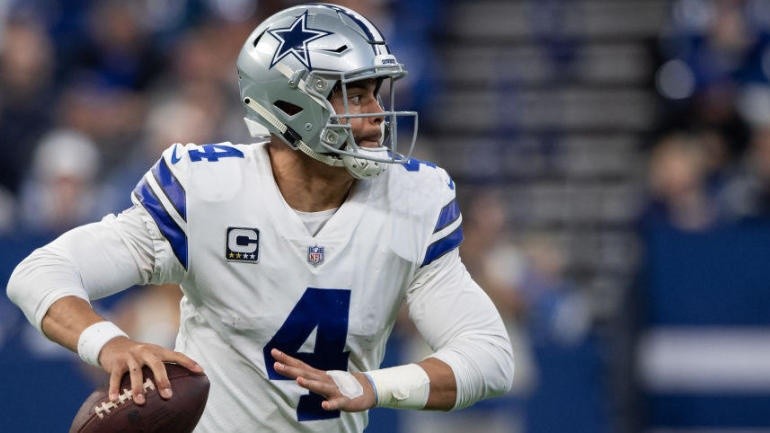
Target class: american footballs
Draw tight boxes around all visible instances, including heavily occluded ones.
[68,365,210,433]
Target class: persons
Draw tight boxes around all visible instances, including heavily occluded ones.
[7,1,517,432]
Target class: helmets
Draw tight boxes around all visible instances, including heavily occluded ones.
[234,2,402,155]
